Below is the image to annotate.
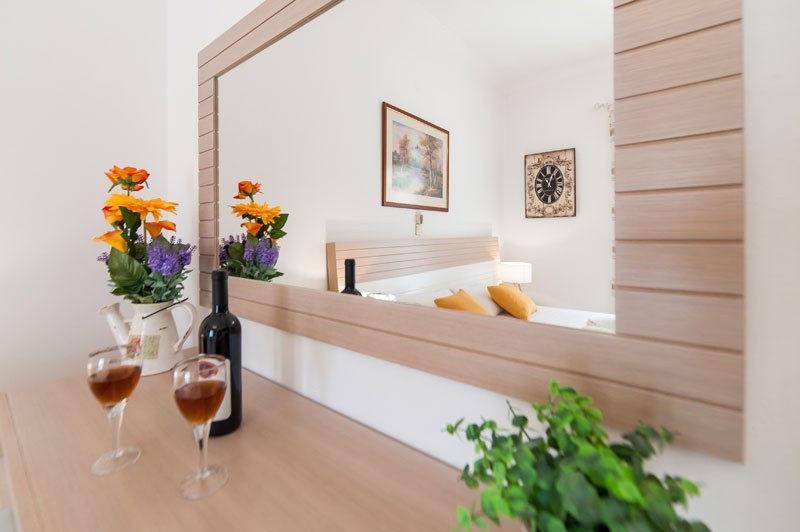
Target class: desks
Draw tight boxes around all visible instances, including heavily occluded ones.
[1,346,540,532]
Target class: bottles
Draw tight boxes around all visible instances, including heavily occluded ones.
[339,258,362,296]
[198,269,243,437]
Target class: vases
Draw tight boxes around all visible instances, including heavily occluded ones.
[101,296,200,381]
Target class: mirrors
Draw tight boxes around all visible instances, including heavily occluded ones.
[195,1,748,467]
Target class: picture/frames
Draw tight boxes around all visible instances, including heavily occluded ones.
[380,102,451,212]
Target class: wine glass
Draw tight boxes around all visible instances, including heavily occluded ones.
[172,353,229,500]
[85,343,144,476]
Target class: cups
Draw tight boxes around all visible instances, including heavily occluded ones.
[367,292,399,302]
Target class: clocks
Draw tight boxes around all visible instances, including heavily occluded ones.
[523,148,576,219]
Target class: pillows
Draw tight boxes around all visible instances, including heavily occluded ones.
[392,284,454,307]
[446,275,506,316]
[487,283,538,322]
[435,288,491,317]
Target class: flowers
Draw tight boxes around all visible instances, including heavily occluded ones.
[91,162,198,307]
[219,180,289,282]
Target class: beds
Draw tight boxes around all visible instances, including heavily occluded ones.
[325,235,616,334]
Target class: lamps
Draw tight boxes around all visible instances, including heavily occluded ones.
[499,261,533,290]
